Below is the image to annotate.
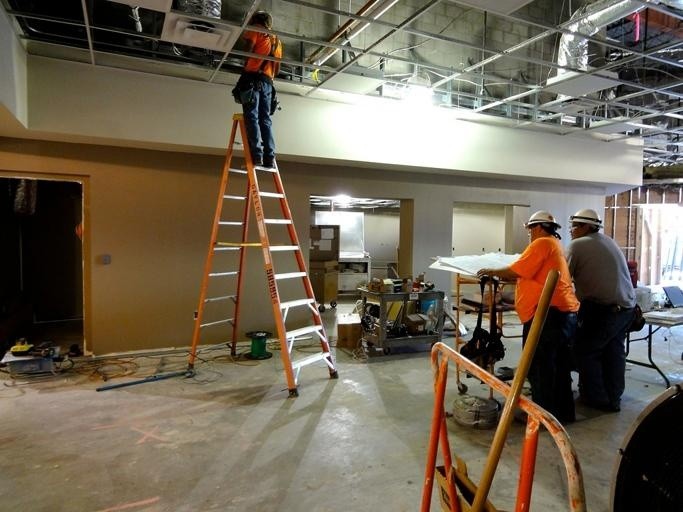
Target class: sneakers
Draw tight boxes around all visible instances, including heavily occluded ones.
[241,159,275,169]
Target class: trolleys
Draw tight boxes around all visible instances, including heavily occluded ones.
[453,274,522,412]
[311,268,337,312]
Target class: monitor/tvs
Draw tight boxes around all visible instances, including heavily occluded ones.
[663,286,683,307]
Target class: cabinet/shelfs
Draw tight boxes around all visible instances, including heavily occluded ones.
[338,252,372,291]
[454,270,575,402]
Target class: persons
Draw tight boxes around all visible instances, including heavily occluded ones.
[562,206,638,420]
[476,209,580,430]
[233,8,282,168]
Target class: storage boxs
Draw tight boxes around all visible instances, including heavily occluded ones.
[310,225,340,261]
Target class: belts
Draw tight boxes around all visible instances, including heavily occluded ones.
[565,312,577,317]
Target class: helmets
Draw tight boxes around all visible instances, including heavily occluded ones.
[568,208,605,228]
[523,210,561,229]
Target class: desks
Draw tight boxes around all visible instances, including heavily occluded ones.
[625,302,683,390]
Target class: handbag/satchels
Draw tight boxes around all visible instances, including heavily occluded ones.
[271,86,278,115]
[232,84,254,104]
[631,304,645,332]
[460,328,504,368]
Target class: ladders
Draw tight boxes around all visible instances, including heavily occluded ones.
[186,112,339,399]
